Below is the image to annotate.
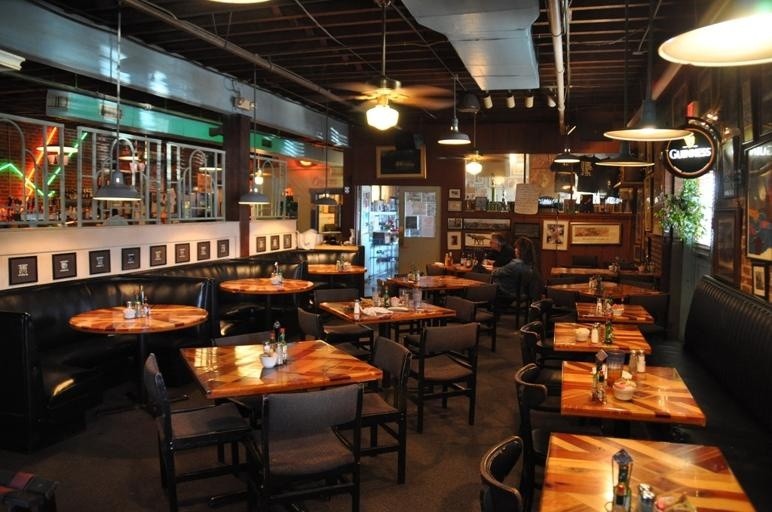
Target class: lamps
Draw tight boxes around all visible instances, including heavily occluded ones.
[93,1,142,203]
[481,87,557,111]
[238,49,272,204]
[594,0,693,171]
[438,71,472,145]
[311,92,340,206]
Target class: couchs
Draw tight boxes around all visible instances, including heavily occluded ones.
[641,272,772,512]
[0,250,366,451]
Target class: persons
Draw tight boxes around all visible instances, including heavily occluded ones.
[280,195,299,220]
[481,234,515,273]
[492,237,538,306]
[580,196,594,213]
[102,208,129,226]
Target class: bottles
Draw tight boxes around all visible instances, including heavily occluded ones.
[612,450,698,511]
[570,318,617,343]
[258,323,291,368]
[122,286,151,318]
[445,249,489,269]
[591,356,643,412]
[351,278,422,319]
[594,295,614,317]
[588,273,603,290]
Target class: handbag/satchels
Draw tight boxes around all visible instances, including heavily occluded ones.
[529,268,545,299]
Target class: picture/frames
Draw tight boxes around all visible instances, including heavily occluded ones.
[670,65,771,301]
[375,141,426,180]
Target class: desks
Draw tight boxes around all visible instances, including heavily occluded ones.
[70,262,755,511]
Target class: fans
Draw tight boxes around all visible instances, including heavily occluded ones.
[317,0,459,110]
